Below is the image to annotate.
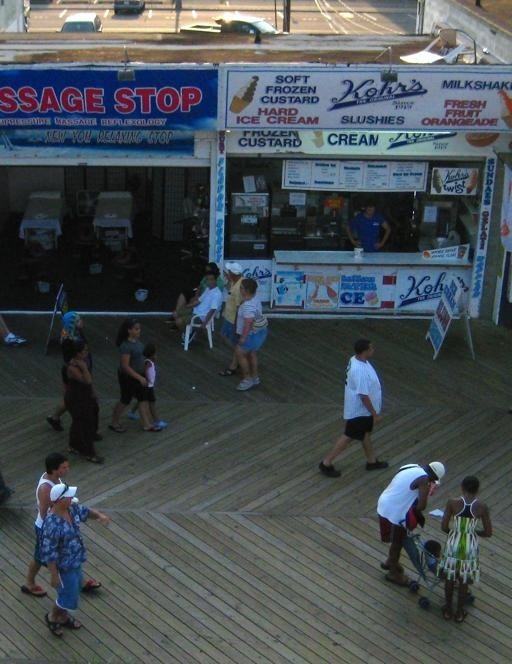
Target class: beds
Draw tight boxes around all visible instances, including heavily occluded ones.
[93,190,134,247]
[19,190,63,248]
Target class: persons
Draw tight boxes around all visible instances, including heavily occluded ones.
[346,202,391,252]
[2,317,27,346]
[376,462,445,586]
[20,453,109,640]
[167,263,272,389]
[45,312,168,463]
[319,339,388,478]
[424,541,442,560]
[2,478,11,504]
[441,476,492,623]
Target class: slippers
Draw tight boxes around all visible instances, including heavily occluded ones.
[82,580,101,591]
[21,585,47,596]
[109,424,127,432]
[381,562,412,586]
[45,613,81,636]
[442,605,467,623]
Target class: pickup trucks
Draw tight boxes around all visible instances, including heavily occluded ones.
[179,14,277,34]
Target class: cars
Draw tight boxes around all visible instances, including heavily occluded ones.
[56,10,104,33]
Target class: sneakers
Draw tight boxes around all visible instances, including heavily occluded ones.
[164,315,179,332]
[8,337,29,345]
[367,461,387,469]
[66,432,104,463]
[47,417,64,431]
[181,329,196,346]
[319,462,341,477]
[219,368,260,390]
[128,411,167,432]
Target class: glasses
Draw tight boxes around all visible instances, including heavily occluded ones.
[60,482,68,495]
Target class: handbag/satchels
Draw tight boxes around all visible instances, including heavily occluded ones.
[252,317,268,331]
[407,509,425,529]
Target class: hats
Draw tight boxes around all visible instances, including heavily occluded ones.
[50,484,77,501]
[429,462,445,484]
[225,262,243,274]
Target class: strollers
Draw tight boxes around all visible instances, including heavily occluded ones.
[402,524,476,608]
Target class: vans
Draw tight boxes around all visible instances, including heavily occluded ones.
[113,0,146,14]
